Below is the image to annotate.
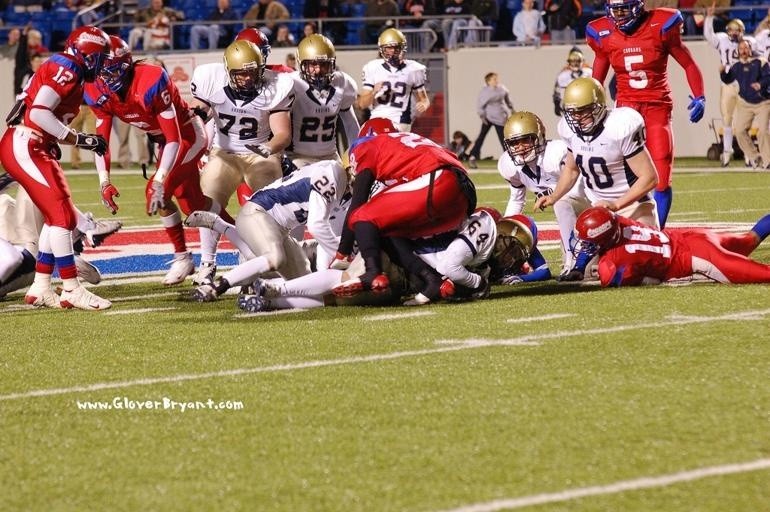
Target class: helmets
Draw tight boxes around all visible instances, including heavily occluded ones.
[568,0,744,70]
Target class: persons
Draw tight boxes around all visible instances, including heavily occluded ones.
[1,0,770,312]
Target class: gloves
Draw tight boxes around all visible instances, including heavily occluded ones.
[245,142,270,159]
[281,157,298,178]
[74,132,108,157]
[329,252,350,270]
[144,180,167,215]
[100,182,120,215]
[687,95,706,124]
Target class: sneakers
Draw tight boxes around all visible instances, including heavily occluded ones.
[331,272,389,298]
[25,210,287,313]
[403,275,456,306]
[722,148,735,167]
